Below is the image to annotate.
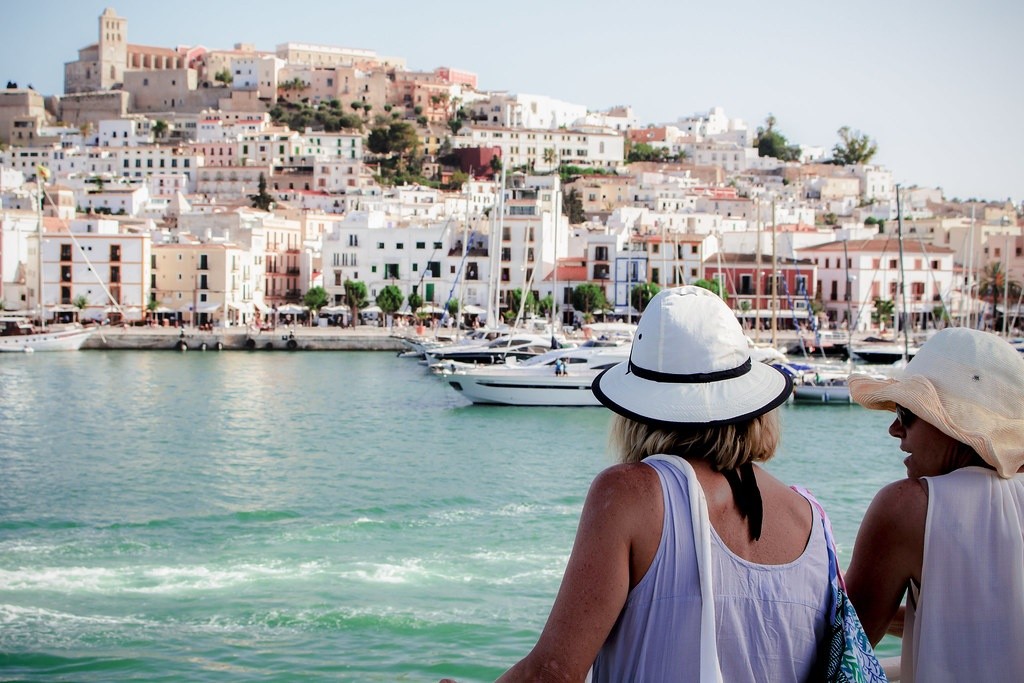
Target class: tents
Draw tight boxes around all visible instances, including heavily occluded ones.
[320,305,351,328]
[278,303,305,325]
[359,301,486,323]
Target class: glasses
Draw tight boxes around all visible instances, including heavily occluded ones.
[895,403,913,427]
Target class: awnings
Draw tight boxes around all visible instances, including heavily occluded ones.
[47,304,80,324]
[256,303,267,311]
[179,303,221,313]
[228,303,255,313]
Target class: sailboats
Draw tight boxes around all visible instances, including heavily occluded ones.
[0,171,127,353]
[388,104,1023,407]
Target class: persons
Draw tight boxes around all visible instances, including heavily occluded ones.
[843,327,1024,683]
[555,356,568,376]
[465,315,479,328]
[395,314,454,328]
[436,288,837,683]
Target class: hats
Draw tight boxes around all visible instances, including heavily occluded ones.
[847,327,1024,479]
[591,286,793,425]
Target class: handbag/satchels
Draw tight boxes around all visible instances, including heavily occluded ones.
[807,488,889,683]
